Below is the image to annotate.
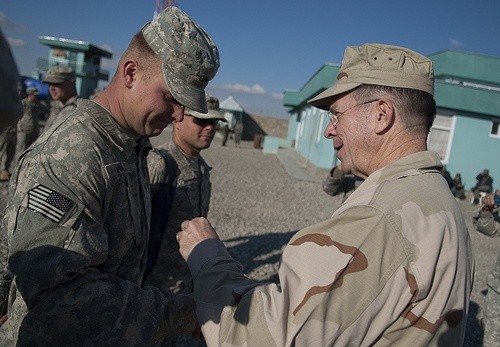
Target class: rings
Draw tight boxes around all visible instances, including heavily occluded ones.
[177,231,182,241]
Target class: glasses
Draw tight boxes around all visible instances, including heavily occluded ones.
[327,99,380,129]
[191,116,218,128]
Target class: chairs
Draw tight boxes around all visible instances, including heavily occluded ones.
[470,189,493,205]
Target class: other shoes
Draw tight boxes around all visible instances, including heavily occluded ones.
[1,169,11,181]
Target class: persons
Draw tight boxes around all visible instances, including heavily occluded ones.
[0,27,78,180]
[472,168,493,205]
[441,162,453,190]
[0,5,220,347]
[253,131,261,148]
[232,119,243,144]
[221,122,231,146]
[451,173,465,199]
[322,164,365,202]
[140,91,227,347]
[472,189,500,238]
[177,42,475,347]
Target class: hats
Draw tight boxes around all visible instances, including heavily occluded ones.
[42,66,76,85]
[26,87,41,96]
[140,6,221,113]
[306,42,435,111]
[185,92,227,122]
